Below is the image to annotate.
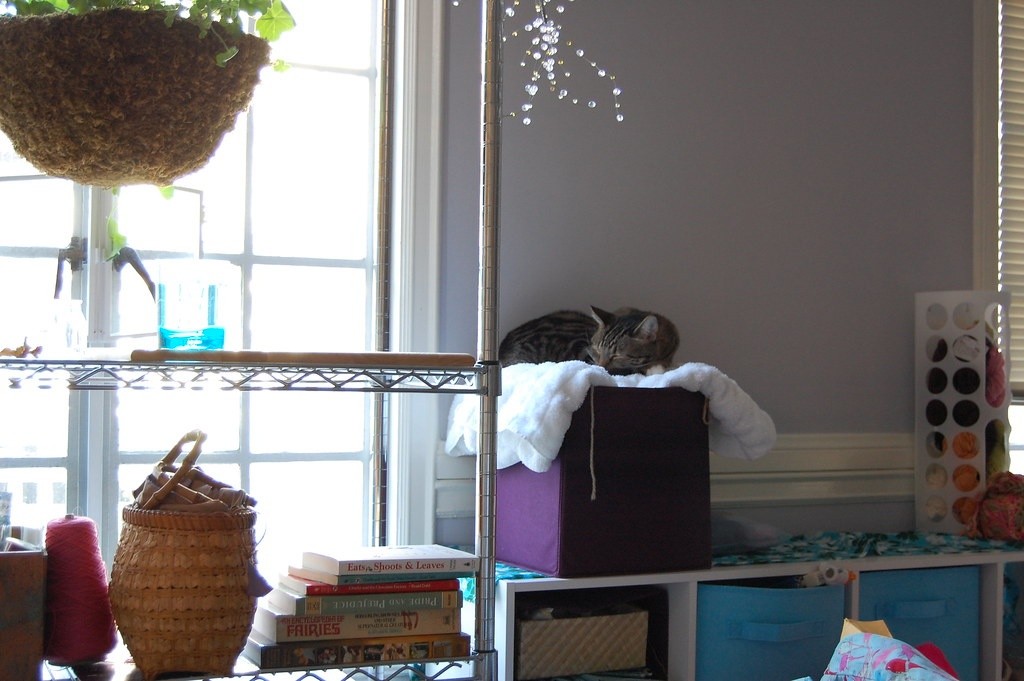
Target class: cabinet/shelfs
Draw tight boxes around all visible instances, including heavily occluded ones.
[0,0,505,681]
[425,536,1024,681]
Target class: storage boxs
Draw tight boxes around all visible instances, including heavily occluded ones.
[445,361,776,577]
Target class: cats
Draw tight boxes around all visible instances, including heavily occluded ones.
[498,305,680,375]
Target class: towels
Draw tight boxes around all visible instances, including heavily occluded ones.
[444,359,779,474]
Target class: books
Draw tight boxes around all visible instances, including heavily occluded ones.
[242,544,480,671]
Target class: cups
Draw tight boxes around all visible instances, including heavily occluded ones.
[154,283,224,351]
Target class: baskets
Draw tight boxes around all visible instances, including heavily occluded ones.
[111,431,257,678]
